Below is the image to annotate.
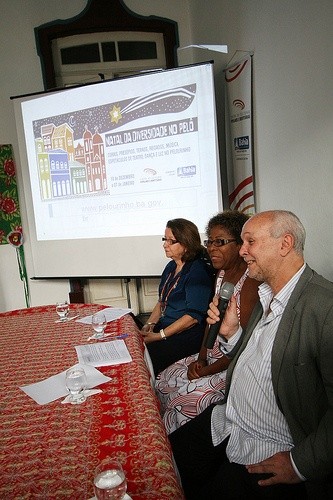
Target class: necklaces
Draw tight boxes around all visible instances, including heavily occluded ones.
[160,274,179,316]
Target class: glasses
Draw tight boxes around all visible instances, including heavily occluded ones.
[162,238,179,245]
[204,239,237,247]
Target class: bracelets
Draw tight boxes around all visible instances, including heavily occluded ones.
[161,329,166,340]
[146,321,156,325]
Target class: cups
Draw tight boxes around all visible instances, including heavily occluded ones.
[93,460,127,500]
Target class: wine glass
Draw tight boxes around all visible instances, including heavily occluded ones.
[56,302,70,322]
[64,366,87,404]
[92,314,107,340]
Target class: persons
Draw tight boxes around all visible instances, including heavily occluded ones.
[167,211,333,500]
[140,219,217,378]
[155,212,264,437]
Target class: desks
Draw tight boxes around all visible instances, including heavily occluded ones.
[0,305,187,500]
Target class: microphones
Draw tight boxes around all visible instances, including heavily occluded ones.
[203,282,234,349]
